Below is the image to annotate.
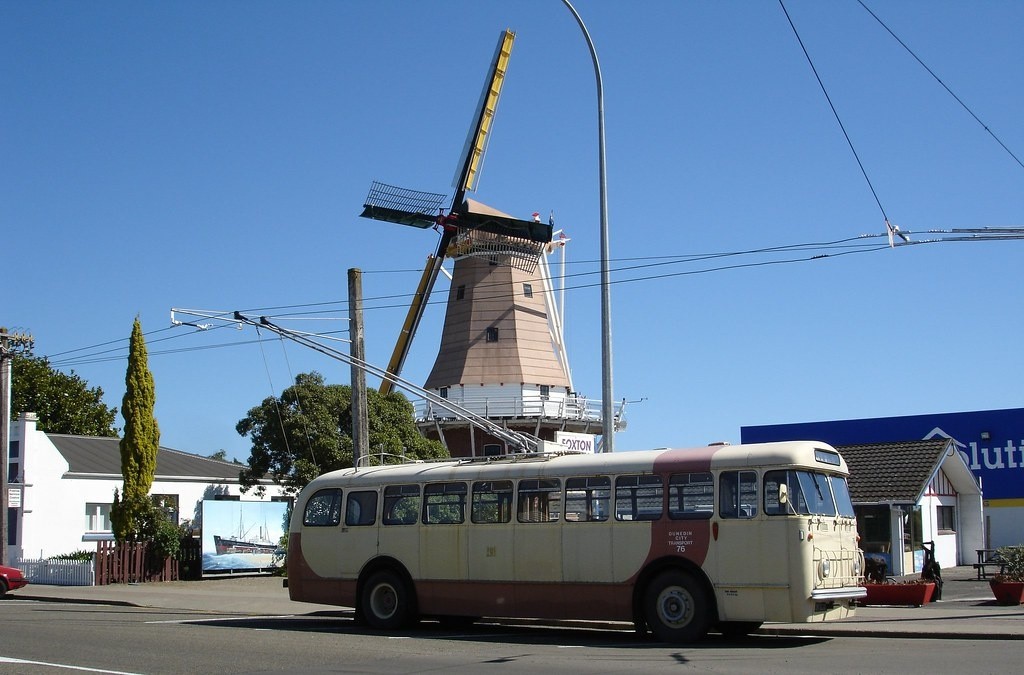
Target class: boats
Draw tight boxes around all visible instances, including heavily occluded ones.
[214,534,278,555]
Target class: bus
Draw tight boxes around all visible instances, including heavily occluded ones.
[283,441,867,647]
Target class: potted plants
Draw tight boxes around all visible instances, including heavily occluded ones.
[990,544,1024,605]
[857,579,935,607]
